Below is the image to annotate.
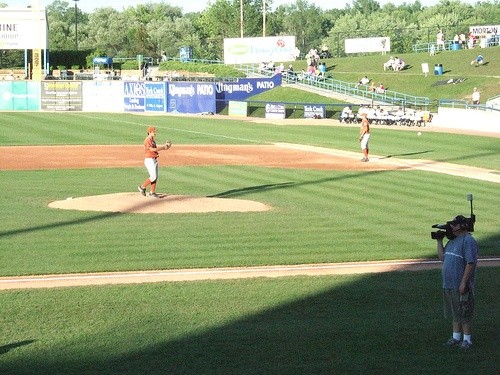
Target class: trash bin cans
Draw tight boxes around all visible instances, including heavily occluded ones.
[435,64,442,75]
[452,41,458,50]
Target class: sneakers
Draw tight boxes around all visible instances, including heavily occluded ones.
[148,193,157,197]
[138,186,146,196]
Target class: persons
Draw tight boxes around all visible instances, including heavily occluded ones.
[138,127,171,200]
[260,60,284,73]
[341,104,431,126]
[368,82,386,94]
[475,52,484,67]
[305,47,326,85]
[468,32,476,49]
[472,87,480,110]
[486,26,498,47]
[321,44,328,55]
[429,44,436,56]
[437,30,446,51]
[459,31,467,48]
[436,214,478,349]
[384,56,406,71]
[359,113,370,162]
[286,64,298,85]
[453,34,458,44]
[356,76,369,87]
[161,50,167,62]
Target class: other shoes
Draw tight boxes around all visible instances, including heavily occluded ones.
[362,158,369,162]
[447,338,461,345]
[459,340,472,349]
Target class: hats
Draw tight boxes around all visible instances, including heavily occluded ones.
[450,215,469,225]
[361,113,367,116]
[147,127,158,132]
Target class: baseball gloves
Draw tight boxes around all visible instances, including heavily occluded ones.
[164,140,171,149]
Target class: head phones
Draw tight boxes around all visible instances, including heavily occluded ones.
[458,215,470,231]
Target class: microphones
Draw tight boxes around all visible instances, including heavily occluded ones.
[456,228,464,231]
[432,225,438,228]
